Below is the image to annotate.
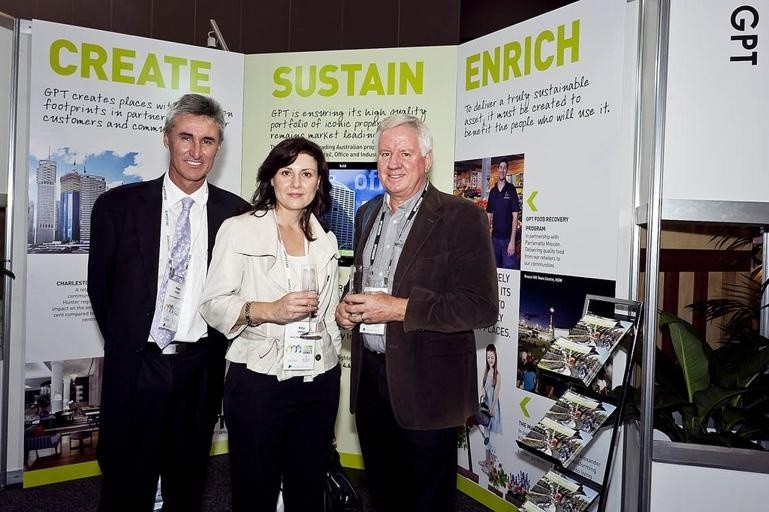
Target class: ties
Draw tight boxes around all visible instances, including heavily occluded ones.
[149,197,195,350]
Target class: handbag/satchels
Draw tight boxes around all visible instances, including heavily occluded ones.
[321,467,362,511]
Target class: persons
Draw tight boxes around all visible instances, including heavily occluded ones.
[85,92,254,511]
[333,114,500,511]
[485,158,520,270]
[198,136,345,511]
[471,342,505,475]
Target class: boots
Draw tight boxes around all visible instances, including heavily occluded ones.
[483,454,497,472]
[479,449,491,465]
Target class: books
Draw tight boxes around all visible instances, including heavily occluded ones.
[514,312,634,512]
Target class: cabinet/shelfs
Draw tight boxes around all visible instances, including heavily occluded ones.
[520,294,642,512]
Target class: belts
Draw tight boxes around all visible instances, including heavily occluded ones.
[364,347,386,366]
[144,342,196,356]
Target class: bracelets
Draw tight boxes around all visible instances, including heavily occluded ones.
[242,300,261,327]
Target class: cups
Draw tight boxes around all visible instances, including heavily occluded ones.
[350,264,371,318]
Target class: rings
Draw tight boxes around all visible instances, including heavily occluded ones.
[358,312,366,323]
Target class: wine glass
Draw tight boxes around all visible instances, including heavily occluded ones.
[301,265,322,339]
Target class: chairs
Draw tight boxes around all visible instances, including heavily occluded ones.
[26,400,99,466]
